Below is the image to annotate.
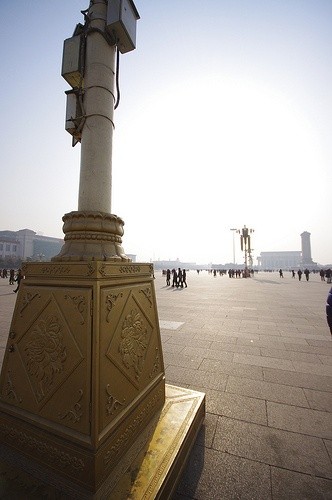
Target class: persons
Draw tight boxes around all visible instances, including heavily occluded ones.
[213,270,226,276]
[229,269,241,278]
[172,268,187,288]
[280,269,283,278]
[320,269,332,282]
[13,269,23,293]
[3,269,8,278]
[304,269,310,281]
[298,269,302,281]
[251,269,253,274]
[292,270,295,278]
[9,269,14,285]
[166,269,171,286]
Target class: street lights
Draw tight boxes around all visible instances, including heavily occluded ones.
[234,225,255,269]
